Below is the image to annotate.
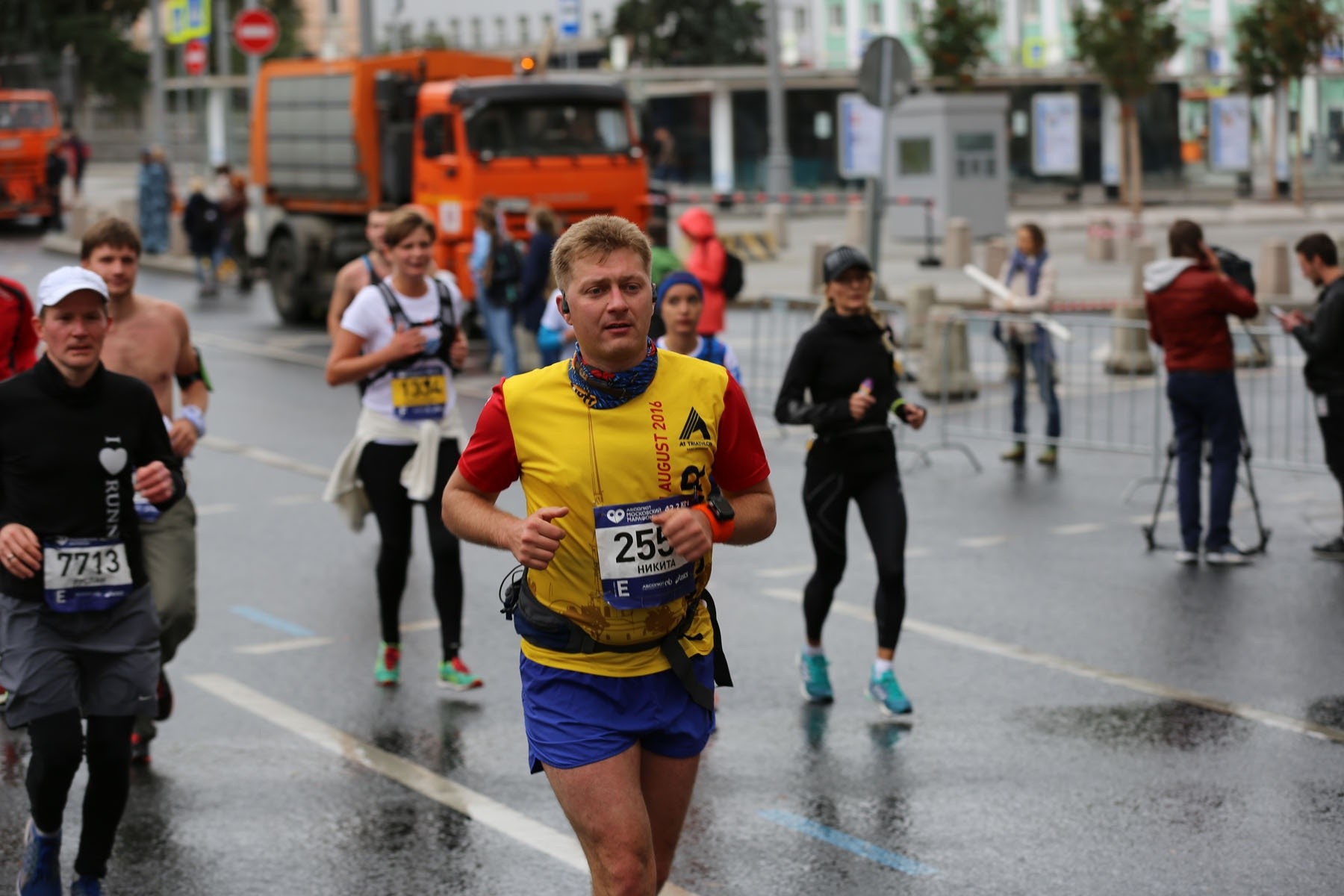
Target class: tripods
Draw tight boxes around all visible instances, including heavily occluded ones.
[1143,431,1267,552]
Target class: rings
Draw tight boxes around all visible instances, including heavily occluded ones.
[6,554,14,560]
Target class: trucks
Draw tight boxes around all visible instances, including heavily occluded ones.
[0,89,63,228]
[252,37,667,342]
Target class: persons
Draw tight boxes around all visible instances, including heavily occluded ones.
[1143,219,1259,566]
[1283,232,1344,562]
[989,222,1062,468]
[441,213,778,896]
[326,194,745,694]
[773,246,927,717]
[0,120,259,896]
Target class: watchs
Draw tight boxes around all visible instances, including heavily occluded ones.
[692,496,735,543]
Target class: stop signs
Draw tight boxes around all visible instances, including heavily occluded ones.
[184,37,207,75]
[232,8,280,57]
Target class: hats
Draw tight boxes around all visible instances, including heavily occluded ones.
[34,265,111,316]
[823,245,873,282]
[466,195,497,228]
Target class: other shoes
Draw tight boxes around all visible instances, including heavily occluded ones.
[1038,450,1055,462]
[70,880,101,896]
[17,817,62,896]
[1205,541,1251,565]
[1176,549,1199,564]
[1311,538,1344,562]
[1002,449,1025,462]
[129,727,156,763]
[156,674,172,719]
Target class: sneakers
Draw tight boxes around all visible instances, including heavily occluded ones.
[376,642,399,687]
[795,649,835,705]
[865,667,913,716]
[436,658,482,692]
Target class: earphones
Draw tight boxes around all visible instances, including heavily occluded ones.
[561,300,570,314]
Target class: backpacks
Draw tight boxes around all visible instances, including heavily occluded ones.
[719,252,744,299]
[491,242,522,282]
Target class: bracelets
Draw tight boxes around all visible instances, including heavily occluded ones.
[177,406,207,437]
[891,398,905,411]
